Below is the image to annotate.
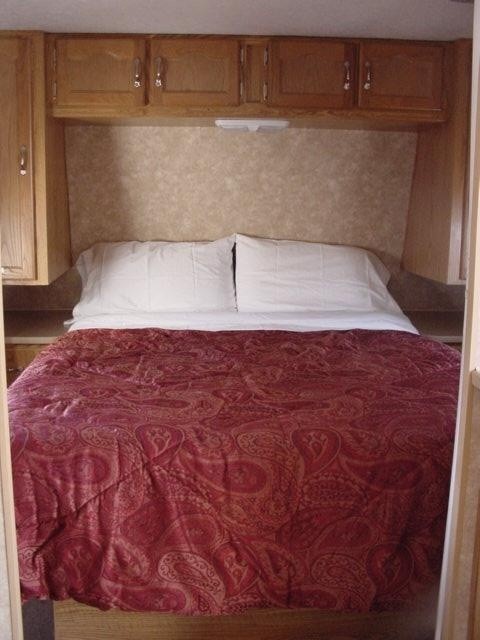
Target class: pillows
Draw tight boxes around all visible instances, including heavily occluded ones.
[65,233,236,330]
[236,235,421,335]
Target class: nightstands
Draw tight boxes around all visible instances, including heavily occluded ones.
[403,311,463,352]
[4,310,73,387]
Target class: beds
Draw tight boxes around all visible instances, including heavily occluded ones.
[6,330,460,640]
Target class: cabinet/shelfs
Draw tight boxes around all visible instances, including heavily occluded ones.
[0,31,71,286]
[45,33,240,121]
[396,39,472,285]
[240,35,455,130]
